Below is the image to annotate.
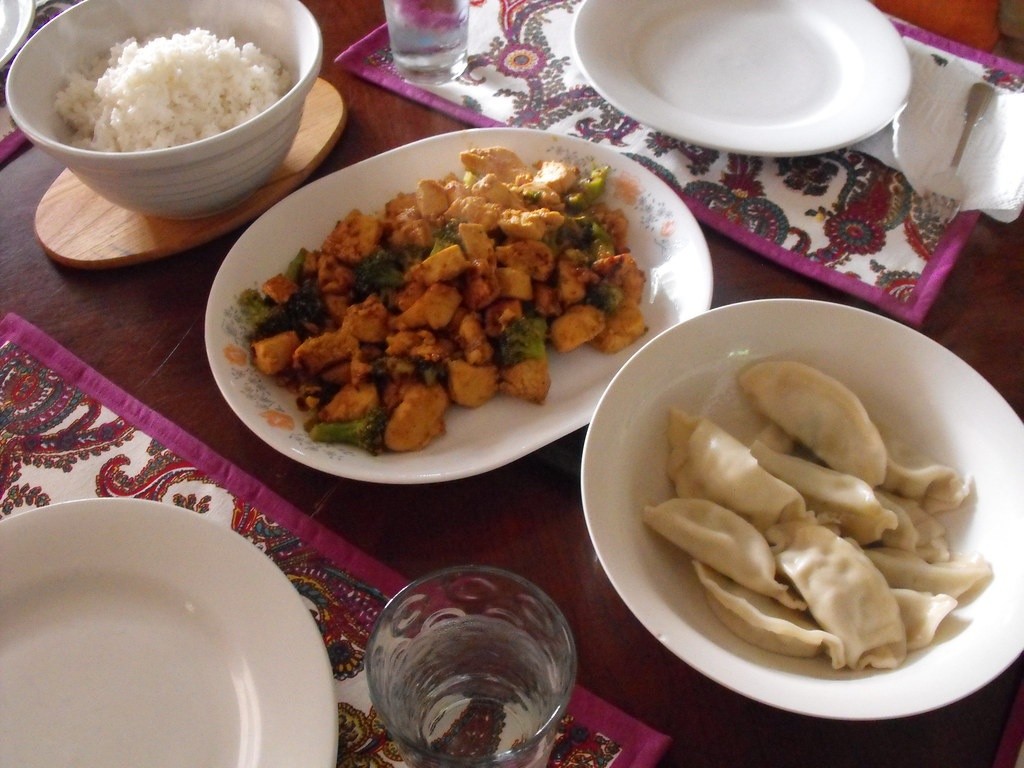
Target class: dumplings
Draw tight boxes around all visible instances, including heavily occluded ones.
[640,361,990,669]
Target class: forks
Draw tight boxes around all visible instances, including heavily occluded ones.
[917,83,994,224]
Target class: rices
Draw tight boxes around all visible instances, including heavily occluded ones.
[55,27,290,150]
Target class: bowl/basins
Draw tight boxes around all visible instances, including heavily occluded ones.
[576,297,1024,718]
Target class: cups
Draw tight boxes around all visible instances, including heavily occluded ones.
[364,562,578,768]
[3,2,323,223]
[382,0,473,87]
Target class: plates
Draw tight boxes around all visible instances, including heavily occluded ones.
[1,499,337,766]
[572,0,914,156]
[202,125,714,487]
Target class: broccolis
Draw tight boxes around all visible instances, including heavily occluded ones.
[242,163,625,454]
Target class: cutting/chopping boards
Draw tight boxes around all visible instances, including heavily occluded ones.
[32,78,347,269]
